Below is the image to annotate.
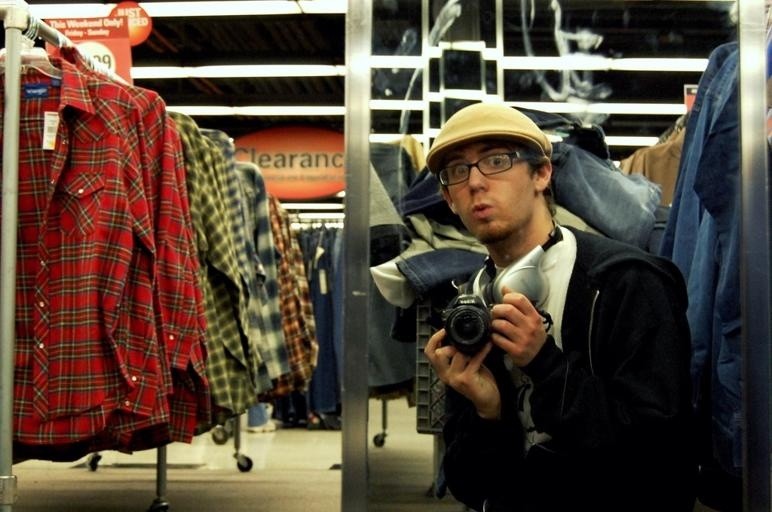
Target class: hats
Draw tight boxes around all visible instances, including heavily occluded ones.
[426,102,553,171]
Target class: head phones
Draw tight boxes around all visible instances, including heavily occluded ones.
[471,227,563,307]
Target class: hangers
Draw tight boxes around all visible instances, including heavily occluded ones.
[20,9,40,53]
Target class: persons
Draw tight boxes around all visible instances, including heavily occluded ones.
[423,101,693,505]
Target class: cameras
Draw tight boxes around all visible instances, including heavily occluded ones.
[436,293,494,355]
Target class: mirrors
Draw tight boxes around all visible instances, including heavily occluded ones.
[347,0,767,509]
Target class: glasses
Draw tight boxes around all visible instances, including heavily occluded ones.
[439,149,545,187]
